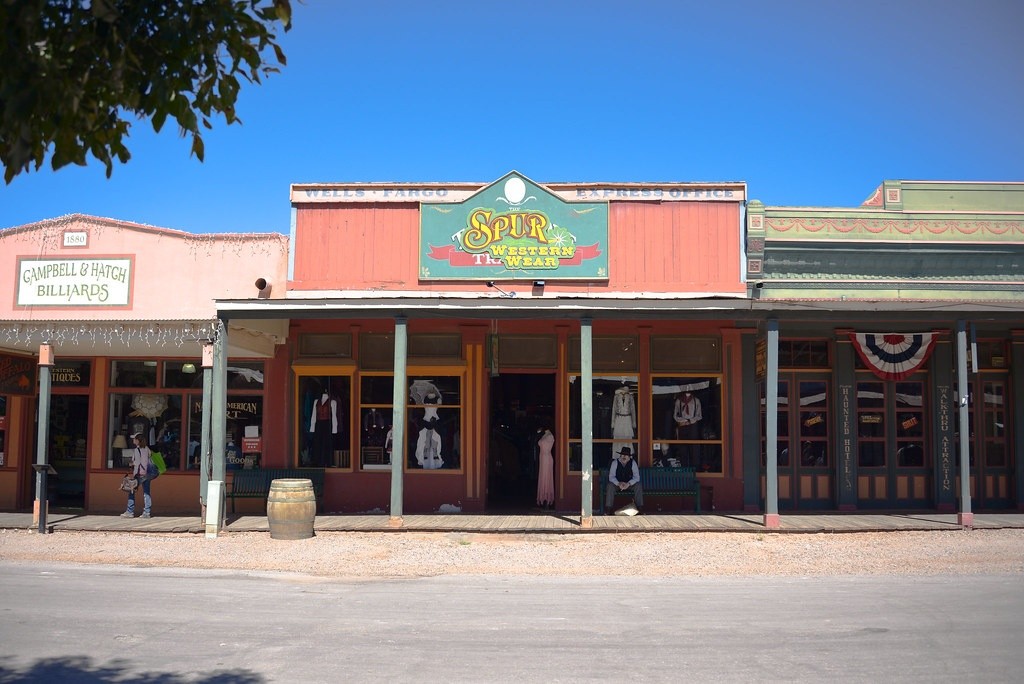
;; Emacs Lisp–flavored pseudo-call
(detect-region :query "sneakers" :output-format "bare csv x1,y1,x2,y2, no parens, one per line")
121,512,135,518
139,514,151,518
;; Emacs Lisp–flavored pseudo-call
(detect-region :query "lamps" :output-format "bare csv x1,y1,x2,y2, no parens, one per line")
182,363,196,373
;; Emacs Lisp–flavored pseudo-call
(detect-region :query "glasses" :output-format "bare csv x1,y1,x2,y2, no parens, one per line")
621,455,628,457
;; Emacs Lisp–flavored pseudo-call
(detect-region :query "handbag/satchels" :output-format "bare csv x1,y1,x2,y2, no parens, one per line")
147,451,166,482
614,499,639,516
118,474,139,494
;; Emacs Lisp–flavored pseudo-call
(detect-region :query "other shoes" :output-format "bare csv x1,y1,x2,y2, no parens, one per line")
636,507,647,516
604,507,612,516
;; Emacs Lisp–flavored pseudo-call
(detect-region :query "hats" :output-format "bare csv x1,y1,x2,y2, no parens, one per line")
130,432,142,439
616,447,634,456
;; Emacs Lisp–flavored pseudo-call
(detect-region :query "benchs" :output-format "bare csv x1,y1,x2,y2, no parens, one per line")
598,466,702,515
226,468,326,514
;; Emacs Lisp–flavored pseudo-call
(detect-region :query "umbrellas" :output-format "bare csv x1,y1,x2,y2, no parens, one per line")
130,384,169,418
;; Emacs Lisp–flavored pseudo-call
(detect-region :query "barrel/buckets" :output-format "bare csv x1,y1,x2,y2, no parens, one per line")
266,478,317,540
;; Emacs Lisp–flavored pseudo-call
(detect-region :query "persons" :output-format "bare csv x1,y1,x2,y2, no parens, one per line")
673,393,703,471
536,430,555,507
309,392,338,467
119,433,155,518
603,446,645,515
364,407,384,446
610,385,637,457
